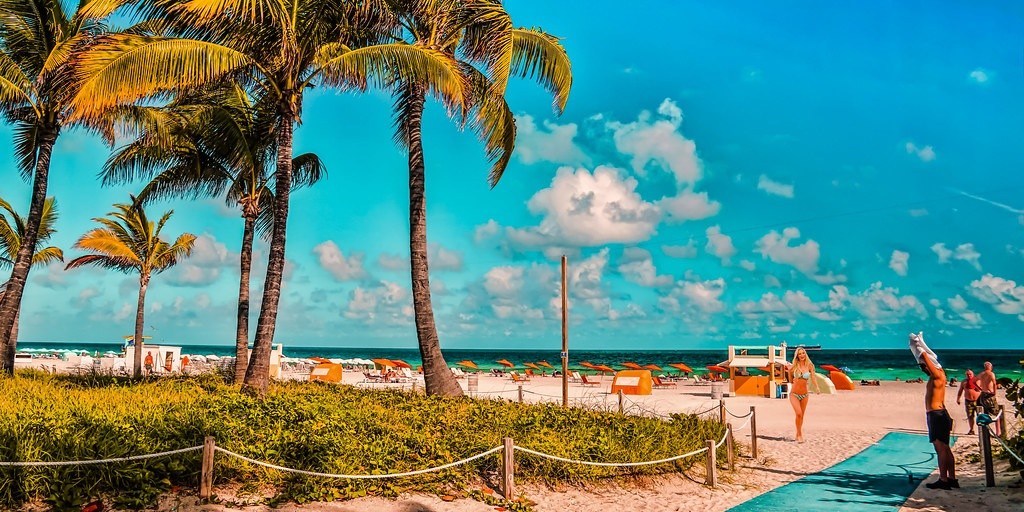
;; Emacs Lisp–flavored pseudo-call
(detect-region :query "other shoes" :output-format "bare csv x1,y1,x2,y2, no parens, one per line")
948,477,960,488
968,431,974,434
926,478,951,490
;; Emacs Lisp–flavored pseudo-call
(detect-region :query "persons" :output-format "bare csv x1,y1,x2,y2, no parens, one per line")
861,376,957,387
956,361,1001,437
385,368,396,382
665,371,689,381
542,369,561,377
788,347,820,444
494,369,507,377
144,351,154,374
909,332,960,491
702,373,723,382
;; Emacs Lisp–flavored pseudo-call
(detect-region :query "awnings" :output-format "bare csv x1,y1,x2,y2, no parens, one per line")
717,357,770,367
774,358,792,367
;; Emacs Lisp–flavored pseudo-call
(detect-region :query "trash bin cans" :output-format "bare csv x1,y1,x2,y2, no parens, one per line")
711,382,723,400
468,375,478,391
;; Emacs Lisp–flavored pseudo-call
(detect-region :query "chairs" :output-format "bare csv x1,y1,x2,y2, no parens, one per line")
363,368,724,388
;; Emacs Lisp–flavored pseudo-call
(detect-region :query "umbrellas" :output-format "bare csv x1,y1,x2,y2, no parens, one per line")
706,365,727,375
496,359,554,372
738,364,840,377
454,360,478,369
578,361,693,376
280,356,412,374
181,353,236,364
19,347,126,356
